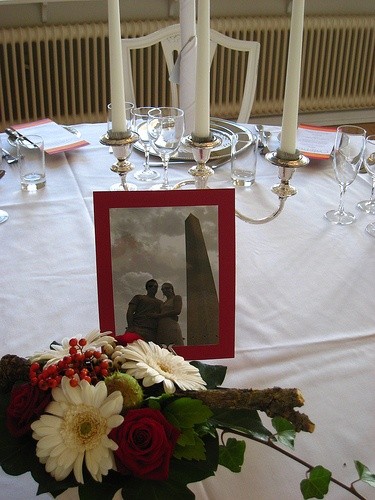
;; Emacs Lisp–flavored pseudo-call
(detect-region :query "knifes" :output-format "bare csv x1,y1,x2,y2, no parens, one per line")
4,128,34,150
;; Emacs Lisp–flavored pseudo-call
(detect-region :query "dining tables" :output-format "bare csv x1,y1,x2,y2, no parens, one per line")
0,119,375,500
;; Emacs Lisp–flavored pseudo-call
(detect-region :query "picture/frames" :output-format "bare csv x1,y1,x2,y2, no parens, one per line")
93,188,235,360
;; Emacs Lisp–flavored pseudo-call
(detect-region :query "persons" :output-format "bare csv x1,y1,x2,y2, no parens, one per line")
126,279,178,341
150,283,184,346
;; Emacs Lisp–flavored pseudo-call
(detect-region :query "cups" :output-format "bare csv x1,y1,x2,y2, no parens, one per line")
231,133,258,186
17,135,47,191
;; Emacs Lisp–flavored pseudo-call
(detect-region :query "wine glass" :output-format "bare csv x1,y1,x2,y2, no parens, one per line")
107,102,134,163
364,219,375,235
325,124,366,225
131,107,161,182
356,135,375,215
148,107,185,190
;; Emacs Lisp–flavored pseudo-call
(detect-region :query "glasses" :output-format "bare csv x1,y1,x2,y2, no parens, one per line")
161,286,172,290
148,285,157,288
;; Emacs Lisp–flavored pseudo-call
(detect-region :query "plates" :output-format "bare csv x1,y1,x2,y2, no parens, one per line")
276,131,351,153
6,126,80,149
131,116,253,160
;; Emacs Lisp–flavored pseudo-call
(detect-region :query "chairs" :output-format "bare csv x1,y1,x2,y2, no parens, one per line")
122,25,260,124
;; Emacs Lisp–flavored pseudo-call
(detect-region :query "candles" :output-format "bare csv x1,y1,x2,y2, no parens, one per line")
195,0,210,137
108,0,126,133
279,0,305,155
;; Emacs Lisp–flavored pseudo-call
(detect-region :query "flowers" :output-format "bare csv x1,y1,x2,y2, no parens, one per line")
0,330,375,500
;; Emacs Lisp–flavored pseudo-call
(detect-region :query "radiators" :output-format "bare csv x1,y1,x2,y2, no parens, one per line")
0,15,375,133
